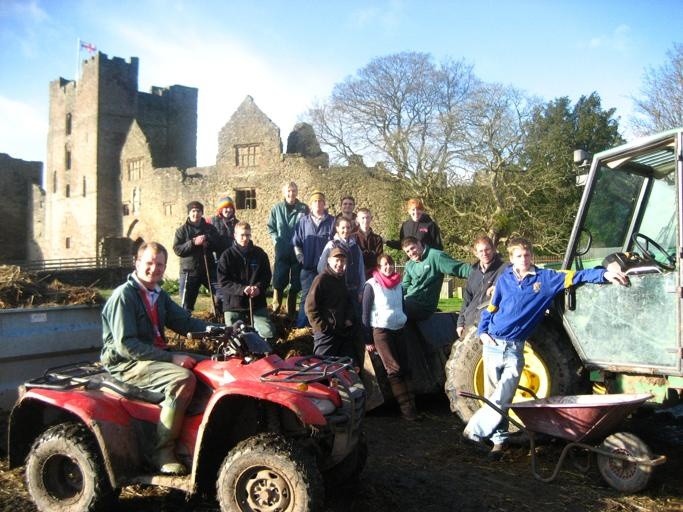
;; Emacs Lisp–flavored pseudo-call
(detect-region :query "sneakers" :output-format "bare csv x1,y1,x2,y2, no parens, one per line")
486,442,507,463
399,406,426,422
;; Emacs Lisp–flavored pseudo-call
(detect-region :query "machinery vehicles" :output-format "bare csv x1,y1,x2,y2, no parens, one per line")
445,127,683,442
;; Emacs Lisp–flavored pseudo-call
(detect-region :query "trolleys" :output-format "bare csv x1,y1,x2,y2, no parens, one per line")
459,387,667,496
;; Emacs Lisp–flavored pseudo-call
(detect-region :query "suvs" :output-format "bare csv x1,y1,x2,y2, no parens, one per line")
9,335,361,508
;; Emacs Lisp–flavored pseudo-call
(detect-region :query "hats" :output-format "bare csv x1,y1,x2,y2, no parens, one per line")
307,188,326,205
329,246,347,257
216,194,236,220
186,199,203,215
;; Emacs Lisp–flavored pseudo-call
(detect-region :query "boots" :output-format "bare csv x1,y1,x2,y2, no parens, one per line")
142,402,190,477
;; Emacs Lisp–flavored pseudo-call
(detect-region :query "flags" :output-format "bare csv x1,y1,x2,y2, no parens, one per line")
74,37,102,55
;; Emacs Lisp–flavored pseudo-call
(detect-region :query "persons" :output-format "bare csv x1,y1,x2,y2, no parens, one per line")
351,208,383,261
217,222,275,339
361,254,428,421
304,247,356,357
173,202,223,313
99,242,245,475
399,236,475,318
206,197,239,244
291,193,336,328
462,236,629,463
382,198,443,260
267,181,310,316
335,197,357,232
457,235,511,338
317,217,366,303
